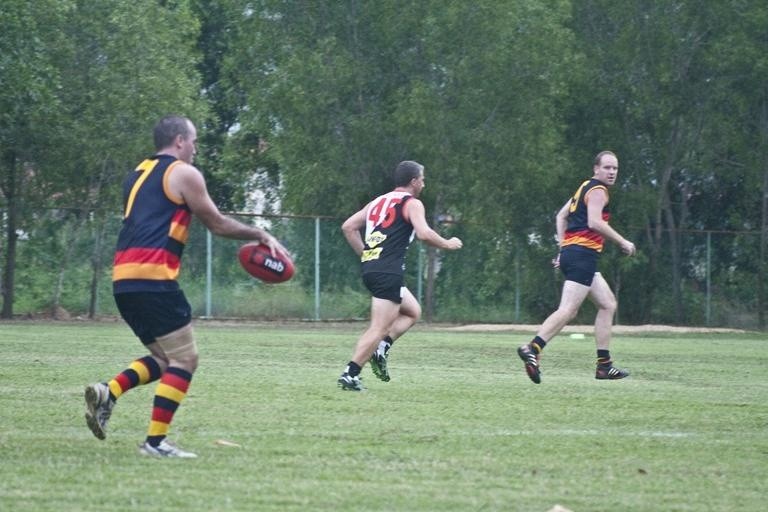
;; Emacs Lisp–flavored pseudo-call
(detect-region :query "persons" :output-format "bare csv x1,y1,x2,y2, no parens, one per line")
335,160,463,391
85,114,290,461
518,151,635,383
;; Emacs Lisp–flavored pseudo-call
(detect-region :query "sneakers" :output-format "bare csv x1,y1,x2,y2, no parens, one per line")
595,356,631,380
517,342,541,384
84,383,115,441
138,441,199,459
337,337,390,391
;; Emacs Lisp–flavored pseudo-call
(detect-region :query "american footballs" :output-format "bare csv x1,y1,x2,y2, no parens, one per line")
239,245,294,284
